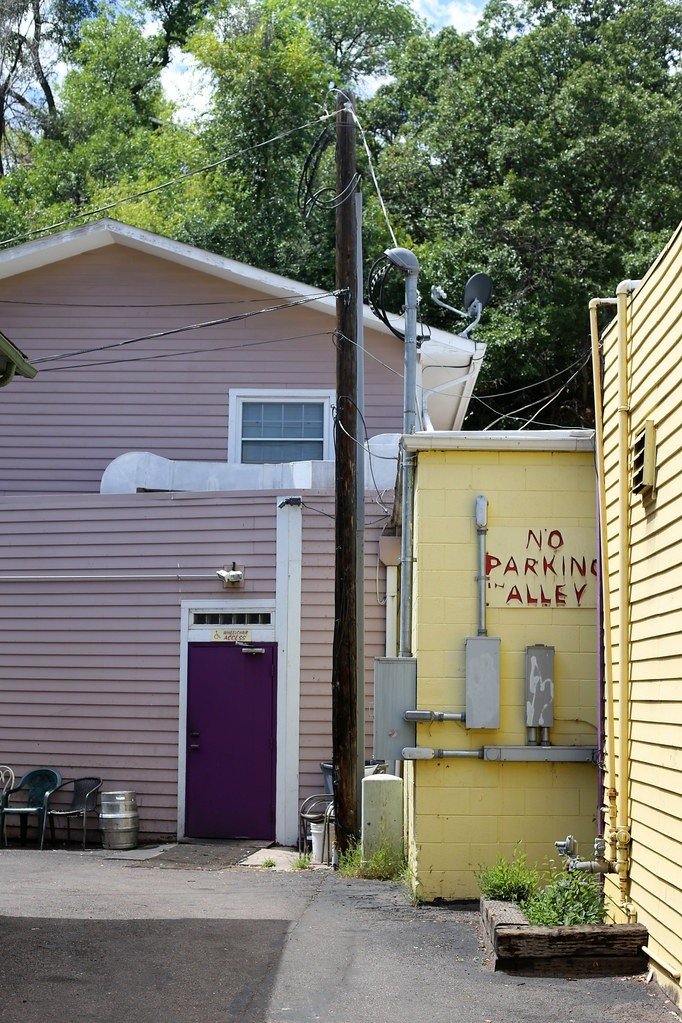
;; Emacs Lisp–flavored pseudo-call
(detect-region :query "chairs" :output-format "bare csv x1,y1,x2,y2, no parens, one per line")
299,794,340,873
0,769,62,850
41,777,103,851
0,765,14,847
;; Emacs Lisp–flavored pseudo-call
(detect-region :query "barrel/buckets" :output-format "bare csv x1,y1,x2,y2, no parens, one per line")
98,789,138,850
310,822,334,862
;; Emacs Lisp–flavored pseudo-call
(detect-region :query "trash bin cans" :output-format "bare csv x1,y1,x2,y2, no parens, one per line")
99,791,140,849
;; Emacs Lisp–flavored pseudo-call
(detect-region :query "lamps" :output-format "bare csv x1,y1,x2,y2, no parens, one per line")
216,569,243,582
